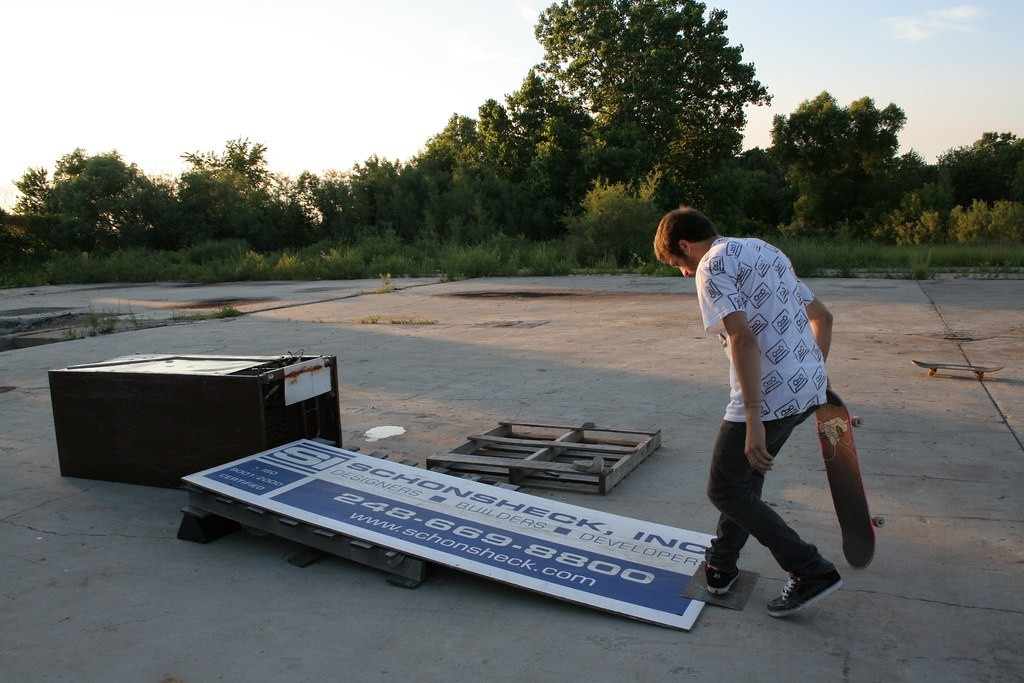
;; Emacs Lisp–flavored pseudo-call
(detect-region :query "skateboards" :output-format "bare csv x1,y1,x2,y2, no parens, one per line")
815,387,886,571
910,360,1004,380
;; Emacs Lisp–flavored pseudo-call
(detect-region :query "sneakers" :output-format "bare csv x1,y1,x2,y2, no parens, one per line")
766,563,843,617
705,563,740,595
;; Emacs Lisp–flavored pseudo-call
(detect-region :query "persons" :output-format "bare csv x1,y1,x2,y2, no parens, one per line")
653,204,843,618
